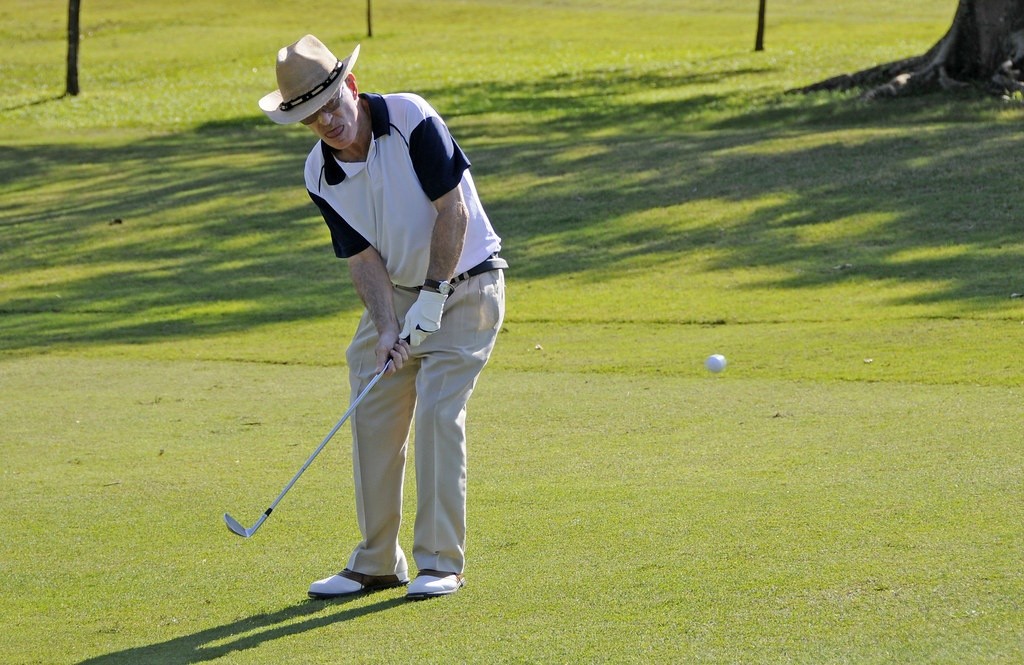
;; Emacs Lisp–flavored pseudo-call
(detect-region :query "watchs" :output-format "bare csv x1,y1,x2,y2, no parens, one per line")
424,279,455,299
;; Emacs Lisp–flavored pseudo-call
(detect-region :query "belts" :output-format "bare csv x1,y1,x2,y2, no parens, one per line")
414,252,510,291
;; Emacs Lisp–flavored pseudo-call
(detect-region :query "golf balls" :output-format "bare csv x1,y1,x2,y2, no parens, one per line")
706,354,726,371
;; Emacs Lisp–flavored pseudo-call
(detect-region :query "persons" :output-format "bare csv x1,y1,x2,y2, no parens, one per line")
260,32,509,600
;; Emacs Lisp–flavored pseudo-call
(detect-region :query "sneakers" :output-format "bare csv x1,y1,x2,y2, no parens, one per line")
406,569,466,600
307,568,411,599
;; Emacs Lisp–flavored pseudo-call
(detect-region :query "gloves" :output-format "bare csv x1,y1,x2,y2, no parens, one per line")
398,290,448,346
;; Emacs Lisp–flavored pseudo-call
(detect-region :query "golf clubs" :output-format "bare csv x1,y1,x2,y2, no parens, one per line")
224,336,412,540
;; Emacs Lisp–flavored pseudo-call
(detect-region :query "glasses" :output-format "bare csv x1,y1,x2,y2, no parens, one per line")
299,84,342,125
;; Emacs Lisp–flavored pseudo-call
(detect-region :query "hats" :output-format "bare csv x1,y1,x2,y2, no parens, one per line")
258,34,361,125
